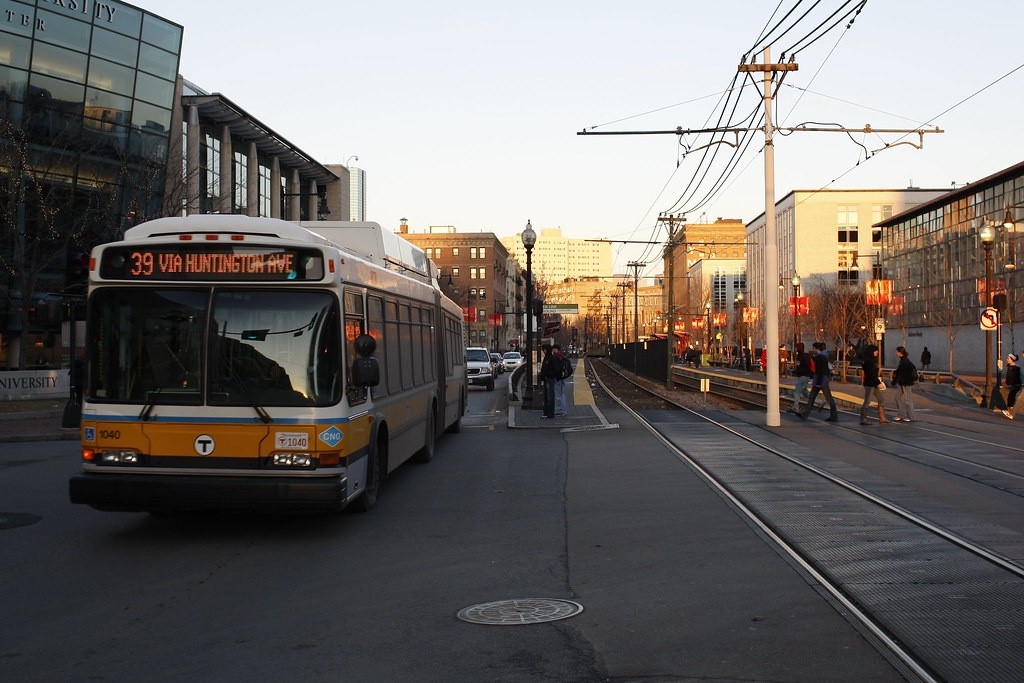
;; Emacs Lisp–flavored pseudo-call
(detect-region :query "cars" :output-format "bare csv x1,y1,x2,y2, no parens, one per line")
502,352,524,371
491,353,503,377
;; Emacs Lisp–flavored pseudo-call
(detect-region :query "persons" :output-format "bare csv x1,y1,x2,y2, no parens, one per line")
860,345,890,425
779,344,788,378
921,347,931,370
786,343,826,414
891,346,917,422
848,346,856,366
796,342,838,422
539,344,560,419
761,344,767,379
1001,351,1024,420
552,344,567,417
683,347,700,369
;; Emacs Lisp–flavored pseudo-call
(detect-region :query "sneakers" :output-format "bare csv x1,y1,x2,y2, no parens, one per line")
1002,411,1013,420
903,417,912,422
892,416,905,421
825,418,838,422
540,415,554,420
795,412,806,421
555,412,567,416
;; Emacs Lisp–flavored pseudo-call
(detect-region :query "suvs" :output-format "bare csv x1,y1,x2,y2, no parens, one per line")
466,347,494,392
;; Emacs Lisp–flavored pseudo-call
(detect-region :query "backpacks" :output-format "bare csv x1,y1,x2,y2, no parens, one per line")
826,358,839,376
808,356,816,375
558,356,572,379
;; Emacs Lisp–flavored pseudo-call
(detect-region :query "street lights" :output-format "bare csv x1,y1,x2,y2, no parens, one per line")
737,291,744,368
979,219,996,407
791,272,800,343
281,191,332,222
706,303,711,354
734,292,752,351
851,253,882,372
779,277,800,362
653,318,657,334
521,219,537,409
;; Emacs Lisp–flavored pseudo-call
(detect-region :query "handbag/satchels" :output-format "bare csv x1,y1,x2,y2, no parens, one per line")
910,362,918,381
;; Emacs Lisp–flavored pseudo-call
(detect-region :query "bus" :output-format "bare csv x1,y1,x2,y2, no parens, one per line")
67,214,467,511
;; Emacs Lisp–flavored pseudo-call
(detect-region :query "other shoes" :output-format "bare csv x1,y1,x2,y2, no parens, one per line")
786,408,796,412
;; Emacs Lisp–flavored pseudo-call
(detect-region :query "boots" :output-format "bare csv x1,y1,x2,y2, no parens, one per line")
860,408,873,425
878,406,892,423
